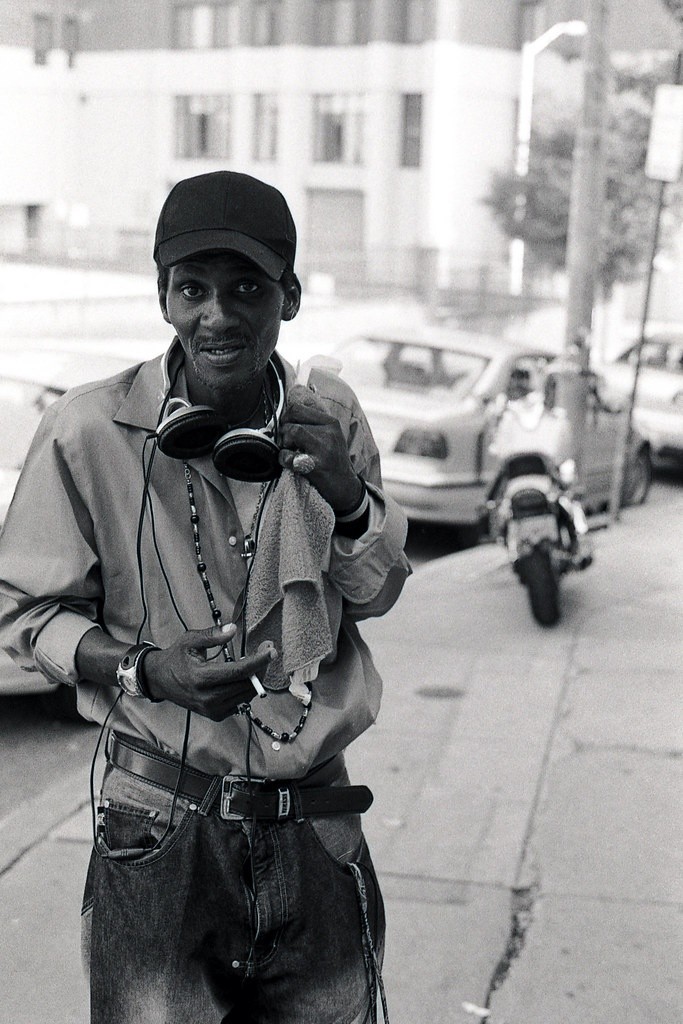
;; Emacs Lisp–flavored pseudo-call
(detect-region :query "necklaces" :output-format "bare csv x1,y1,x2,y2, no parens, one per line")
184,387,312,742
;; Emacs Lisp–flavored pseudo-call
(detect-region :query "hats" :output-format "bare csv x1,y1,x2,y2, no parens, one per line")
154,170,297,280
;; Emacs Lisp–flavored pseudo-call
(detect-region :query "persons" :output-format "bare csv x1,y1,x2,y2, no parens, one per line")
0,170,414,1024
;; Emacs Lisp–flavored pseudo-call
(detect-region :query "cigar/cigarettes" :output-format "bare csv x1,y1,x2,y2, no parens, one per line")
240,657,268,698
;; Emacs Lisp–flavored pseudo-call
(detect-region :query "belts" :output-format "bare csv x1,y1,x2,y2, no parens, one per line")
108,735,374,820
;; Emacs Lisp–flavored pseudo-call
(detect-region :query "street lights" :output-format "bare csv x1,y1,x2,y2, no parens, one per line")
508,21,590,289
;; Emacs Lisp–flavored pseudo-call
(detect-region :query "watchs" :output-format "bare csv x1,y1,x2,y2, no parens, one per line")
115,640,165,703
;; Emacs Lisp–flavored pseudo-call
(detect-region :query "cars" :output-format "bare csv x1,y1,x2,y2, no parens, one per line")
329,324,652,554
605,321,682,459
0,351,157,724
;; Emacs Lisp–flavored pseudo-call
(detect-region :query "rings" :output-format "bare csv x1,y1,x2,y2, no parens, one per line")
292,453,315,476
238,702,251,713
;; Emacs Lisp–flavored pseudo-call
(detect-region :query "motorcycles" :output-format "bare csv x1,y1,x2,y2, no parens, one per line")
472,368,593,627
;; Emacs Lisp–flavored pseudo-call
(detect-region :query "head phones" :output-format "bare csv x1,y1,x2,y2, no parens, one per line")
156,352,285,482
